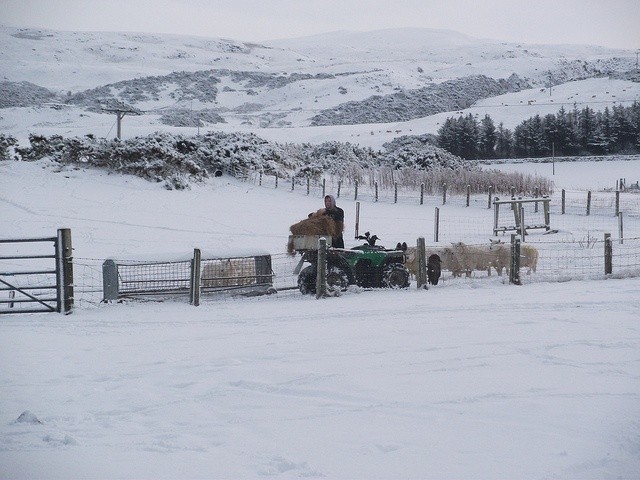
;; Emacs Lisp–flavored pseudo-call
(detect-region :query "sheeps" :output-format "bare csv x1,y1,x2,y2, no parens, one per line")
488,238,539,275
451,241,493,278
200,258,236,286
404,247,438,280
442,247,472,277
432,248,448,271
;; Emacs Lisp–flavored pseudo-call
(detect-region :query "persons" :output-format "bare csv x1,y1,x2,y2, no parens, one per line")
306,191,346,249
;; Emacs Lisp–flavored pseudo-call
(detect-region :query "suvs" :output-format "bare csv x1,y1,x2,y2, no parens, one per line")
298,231,410,296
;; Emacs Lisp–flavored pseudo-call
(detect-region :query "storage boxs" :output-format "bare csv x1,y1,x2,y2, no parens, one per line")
288,234,332,250
